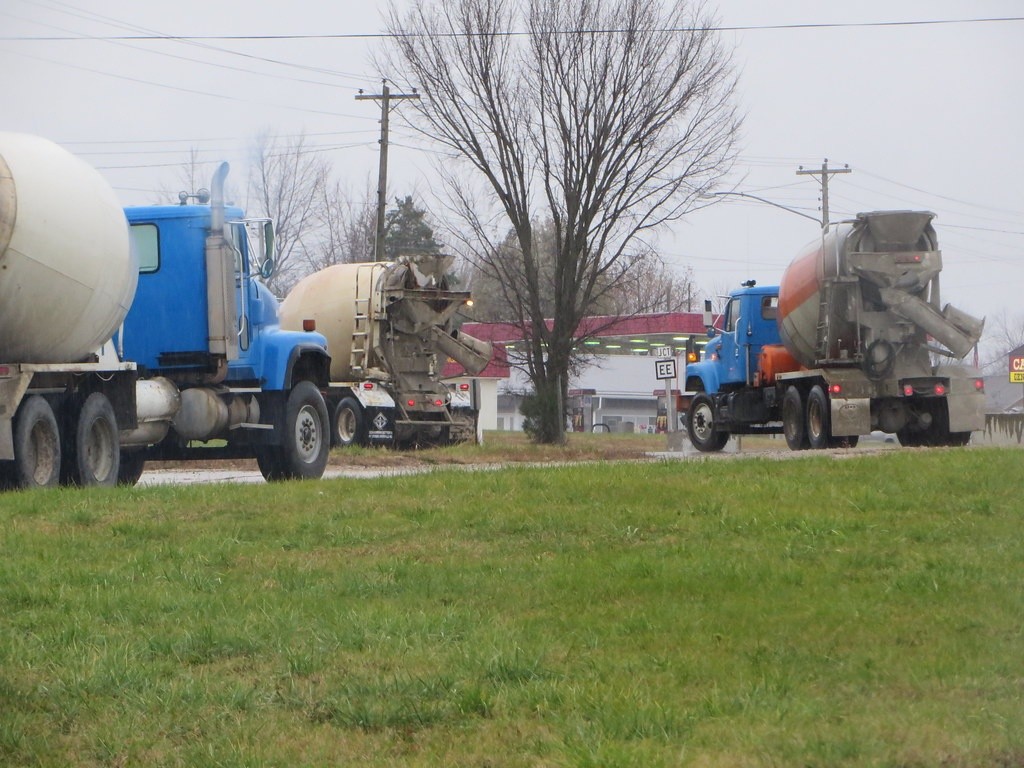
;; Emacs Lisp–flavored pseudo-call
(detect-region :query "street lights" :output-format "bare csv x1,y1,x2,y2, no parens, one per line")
702,189,831,234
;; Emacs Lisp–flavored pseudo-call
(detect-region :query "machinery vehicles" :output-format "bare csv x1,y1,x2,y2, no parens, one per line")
684,210,988,454
0,122,333,496
279,252,494,448
